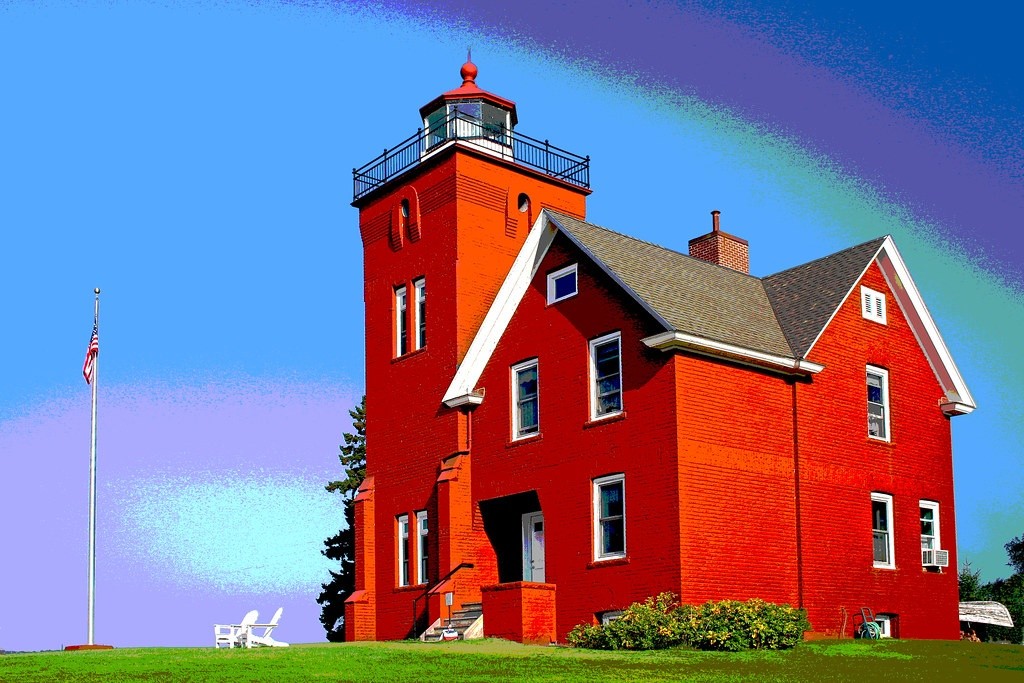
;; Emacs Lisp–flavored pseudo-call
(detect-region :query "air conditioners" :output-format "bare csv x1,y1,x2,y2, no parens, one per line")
921,548,949,567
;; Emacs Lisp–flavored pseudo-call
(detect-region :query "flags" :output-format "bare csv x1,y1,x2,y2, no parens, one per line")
82,315,99,386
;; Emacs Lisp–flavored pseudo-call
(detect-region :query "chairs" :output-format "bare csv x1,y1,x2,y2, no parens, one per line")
214,608,290,648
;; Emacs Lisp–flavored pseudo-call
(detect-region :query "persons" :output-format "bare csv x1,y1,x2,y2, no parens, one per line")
960,628,981,642
